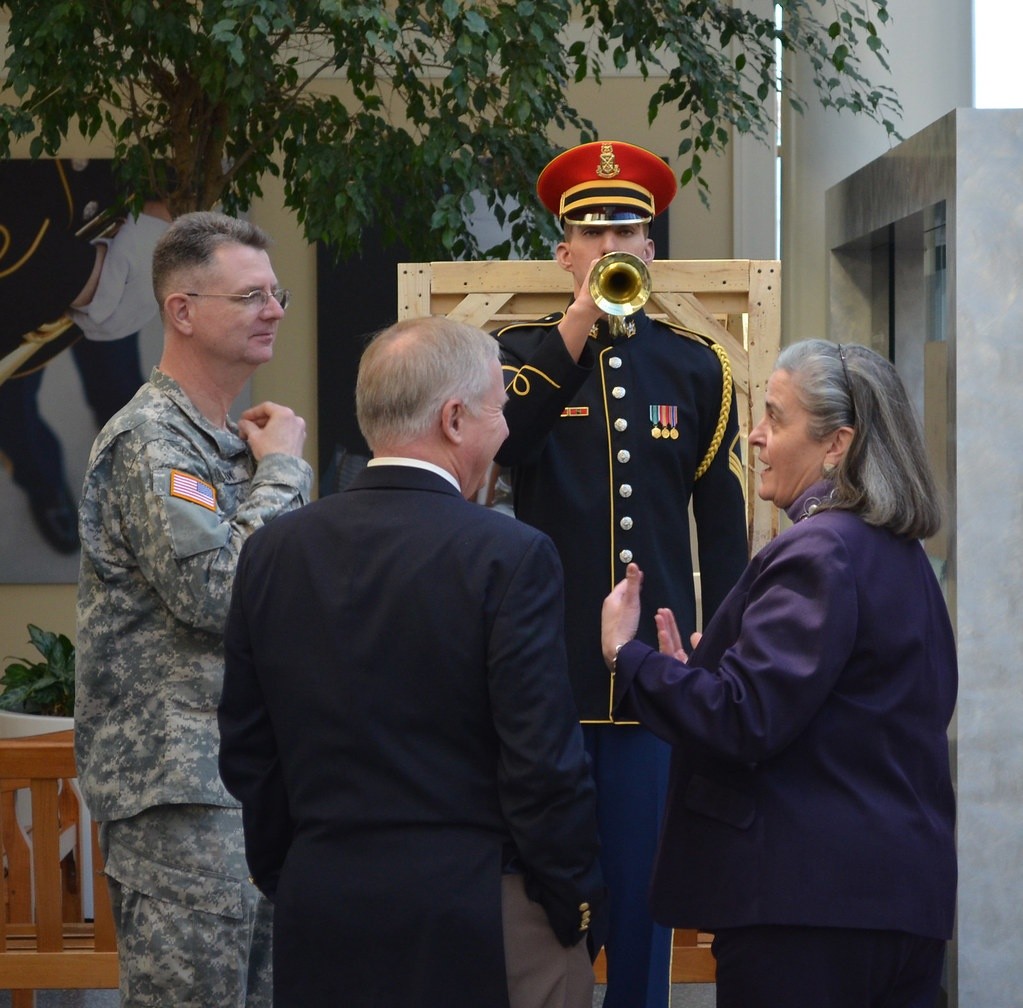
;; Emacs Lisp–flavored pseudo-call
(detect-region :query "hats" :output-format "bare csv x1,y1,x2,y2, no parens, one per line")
536,140,677,225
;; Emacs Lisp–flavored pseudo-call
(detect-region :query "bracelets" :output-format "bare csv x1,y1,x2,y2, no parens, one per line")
612,638,628,684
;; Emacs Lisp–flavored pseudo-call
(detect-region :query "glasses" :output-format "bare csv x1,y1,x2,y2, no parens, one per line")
183,288,292,313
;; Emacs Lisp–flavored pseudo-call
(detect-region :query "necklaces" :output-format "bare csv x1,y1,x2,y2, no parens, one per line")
796,486,839,523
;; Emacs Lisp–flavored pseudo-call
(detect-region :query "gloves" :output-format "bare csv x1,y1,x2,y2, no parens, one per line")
73,211,171,342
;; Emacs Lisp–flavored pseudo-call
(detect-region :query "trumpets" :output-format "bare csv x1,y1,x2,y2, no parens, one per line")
588,251,654,317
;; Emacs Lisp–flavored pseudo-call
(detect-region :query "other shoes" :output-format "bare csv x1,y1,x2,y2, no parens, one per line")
29,477,79,554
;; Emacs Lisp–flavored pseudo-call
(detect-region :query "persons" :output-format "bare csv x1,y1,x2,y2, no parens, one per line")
0,160,195,558
601,339,959,1008
57,212,322,1008
215,314,604,1008
485,139,752,1008
